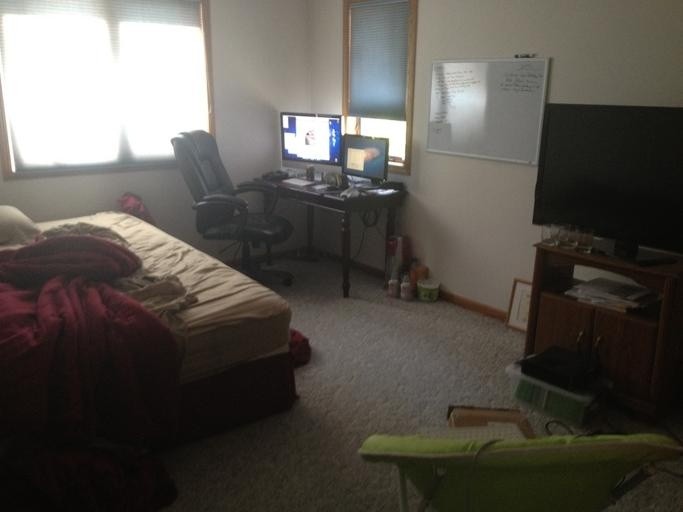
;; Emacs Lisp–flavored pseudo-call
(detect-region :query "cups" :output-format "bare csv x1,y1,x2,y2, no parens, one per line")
541,221,593,253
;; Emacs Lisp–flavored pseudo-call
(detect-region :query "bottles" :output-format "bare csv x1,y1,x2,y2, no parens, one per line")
388,270,412,300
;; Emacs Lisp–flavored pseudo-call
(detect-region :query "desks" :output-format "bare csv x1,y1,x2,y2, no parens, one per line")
254,171,409,297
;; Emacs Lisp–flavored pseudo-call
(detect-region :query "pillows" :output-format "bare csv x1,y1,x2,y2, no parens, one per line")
1,205,44,247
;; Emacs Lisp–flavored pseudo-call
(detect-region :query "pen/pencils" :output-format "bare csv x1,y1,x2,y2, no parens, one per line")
515,54,535,58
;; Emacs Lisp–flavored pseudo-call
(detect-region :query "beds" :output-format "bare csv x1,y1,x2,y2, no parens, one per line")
1,209,293,465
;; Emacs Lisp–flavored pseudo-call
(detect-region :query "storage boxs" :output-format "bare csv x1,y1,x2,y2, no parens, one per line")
507,353,594,426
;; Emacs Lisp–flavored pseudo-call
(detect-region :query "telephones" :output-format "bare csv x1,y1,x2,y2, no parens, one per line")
262,170,287,181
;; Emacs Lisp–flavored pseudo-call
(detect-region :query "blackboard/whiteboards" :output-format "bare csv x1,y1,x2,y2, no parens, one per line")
424,57,551,165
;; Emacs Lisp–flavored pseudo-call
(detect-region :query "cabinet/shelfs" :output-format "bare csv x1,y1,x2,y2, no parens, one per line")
524,237,682,426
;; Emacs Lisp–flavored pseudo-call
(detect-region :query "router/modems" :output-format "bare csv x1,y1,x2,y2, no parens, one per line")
262,171,285,181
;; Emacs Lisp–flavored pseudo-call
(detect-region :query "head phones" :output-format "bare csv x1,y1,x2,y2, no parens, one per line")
323,172,349,191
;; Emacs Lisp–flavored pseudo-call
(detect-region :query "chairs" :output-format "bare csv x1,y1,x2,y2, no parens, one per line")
357,421,683,512
170,129,294,286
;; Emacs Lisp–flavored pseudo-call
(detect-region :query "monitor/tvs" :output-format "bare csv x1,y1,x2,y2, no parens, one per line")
531,102,683,268
279,111,344,185
342,133,390,190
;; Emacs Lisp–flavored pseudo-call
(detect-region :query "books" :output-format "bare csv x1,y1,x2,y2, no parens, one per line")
564,276,662,313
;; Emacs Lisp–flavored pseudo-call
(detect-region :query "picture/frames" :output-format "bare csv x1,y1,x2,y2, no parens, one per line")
505,279,532,333
